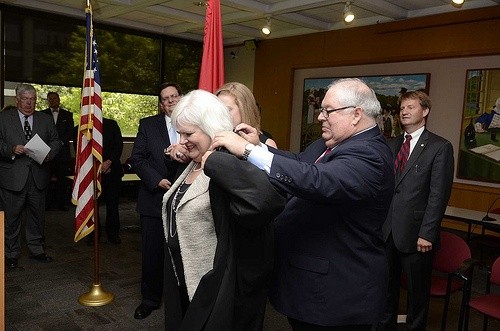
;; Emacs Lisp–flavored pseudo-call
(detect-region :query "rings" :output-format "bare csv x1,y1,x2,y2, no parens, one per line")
176,151,181,158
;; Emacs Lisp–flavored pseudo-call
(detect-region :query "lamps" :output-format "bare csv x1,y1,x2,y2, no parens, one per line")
343,1,354,23
261,13,272,35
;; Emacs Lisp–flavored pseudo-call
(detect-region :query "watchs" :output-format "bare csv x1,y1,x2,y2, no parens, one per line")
242,143,255,160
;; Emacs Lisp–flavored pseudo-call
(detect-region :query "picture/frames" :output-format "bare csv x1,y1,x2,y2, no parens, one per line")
289,52,500,195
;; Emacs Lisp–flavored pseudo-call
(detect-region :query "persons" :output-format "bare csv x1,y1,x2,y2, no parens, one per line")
208,77,396,331
158,87,290,331
132,81,183,320
305,81,326,124
389,90,455,331
376,103,396,140
214,81,279,150
0,83,124,269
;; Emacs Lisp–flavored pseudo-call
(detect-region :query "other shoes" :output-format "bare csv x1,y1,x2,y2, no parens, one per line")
87,236,95,246
108,237,121,244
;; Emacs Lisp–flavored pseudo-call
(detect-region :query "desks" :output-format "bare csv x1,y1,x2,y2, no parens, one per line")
65,174,142,185
443,206,500,270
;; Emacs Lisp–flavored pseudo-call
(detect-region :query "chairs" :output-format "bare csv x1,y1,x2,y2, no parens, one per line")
466,257,500,331
399,232,472,331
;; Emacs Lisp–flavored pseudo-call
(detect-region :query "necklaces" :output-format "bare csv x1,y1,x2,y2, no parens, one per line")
168,163,202,239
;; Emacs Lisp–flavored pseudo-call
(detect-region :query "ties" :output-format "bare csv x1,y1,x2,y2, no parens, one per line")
169,126,178,145
53,111,57,125
23,115,32,141
393,135,412,177
315,149,331,164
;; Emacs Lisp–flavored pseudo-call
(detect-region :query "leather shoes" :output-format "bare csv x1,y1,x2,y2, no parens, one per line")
61,204,70,211
133,303,160,319
6,258,16,268
29,254,53,262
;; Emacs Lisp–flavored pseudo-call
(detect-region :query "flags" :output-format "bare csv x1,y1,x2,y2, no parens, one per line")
197,0,225,93
70,3,103,243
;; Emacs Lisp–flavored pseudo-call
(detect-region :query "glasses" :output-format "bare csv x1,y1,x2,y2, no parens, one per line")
319,106,356,118
